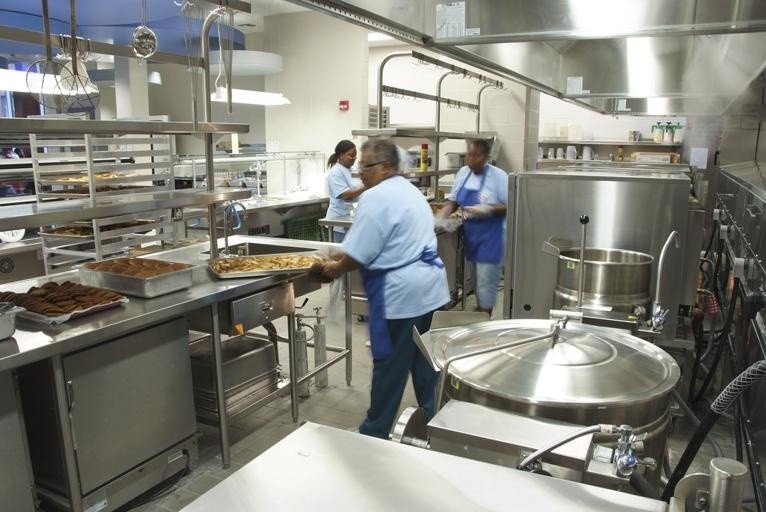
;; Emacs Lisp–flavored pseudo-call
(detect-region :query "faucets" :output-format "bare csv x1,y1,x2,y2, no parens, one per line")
224,202,249,254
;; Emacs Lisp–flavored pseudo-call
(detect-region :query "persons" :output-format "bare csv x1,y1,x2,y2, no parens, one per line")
432,140,508,316
325,140,364,242
322,138,450,438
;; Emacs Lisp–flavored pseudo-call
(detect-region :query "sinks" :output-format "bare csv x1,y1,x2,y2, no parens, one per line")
202,243,318,256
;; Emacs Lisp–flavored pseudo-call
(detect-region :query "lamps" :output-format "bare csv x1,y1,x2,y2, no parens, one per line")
210,19,291,106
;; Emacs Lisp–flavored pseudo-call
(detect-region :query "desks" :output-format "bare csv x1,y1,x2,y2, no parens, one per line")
177,422,669,512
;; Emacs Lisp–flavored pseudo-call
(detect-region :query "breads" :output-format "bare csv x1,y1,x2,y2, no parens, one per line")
85,258,190,279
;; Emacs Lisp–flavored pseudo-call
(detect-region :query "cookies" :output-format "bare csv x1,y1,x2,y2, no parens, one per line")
0,278,122,317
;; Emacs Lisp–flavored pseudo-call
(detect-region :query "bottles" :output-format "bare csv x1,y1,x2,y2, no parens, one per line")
617,145,624,161
421,144,428,172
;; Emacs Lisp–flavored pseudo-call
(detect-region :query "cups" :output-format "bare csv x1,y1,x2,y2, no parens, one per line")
538,146,594,160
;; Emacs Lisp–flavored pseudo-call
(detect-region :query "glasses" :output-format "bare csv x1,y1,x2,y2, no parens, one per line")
356,161,386,169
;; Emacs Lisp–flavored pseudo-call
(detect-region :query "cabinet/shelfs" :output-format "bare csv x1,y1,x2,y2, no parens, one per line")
19,315,198,512
539,139,690,172
0,117,252,289
353,128,497,202
